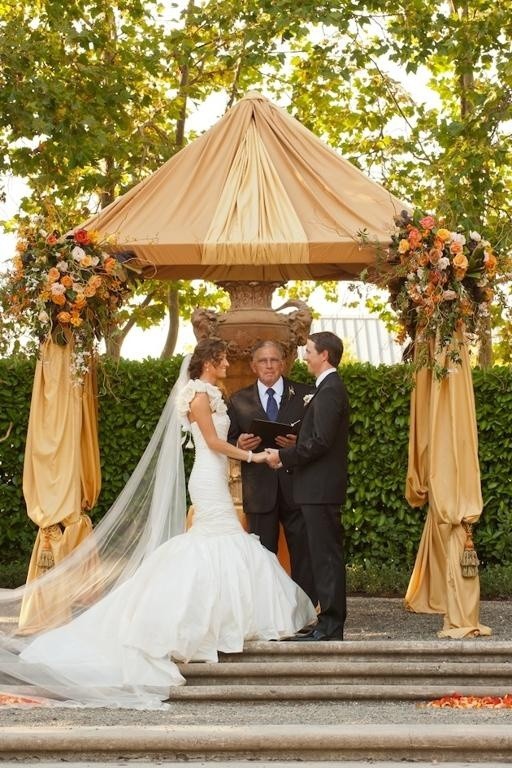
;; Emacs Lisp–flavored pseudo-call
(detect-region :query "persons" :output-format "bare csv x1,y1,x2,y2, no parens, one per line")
265,332,350,641
178,336,279,642
227,339,317,608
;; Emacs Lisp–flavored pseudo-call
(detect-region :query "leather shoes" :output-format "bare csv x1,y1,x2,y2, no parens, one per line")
292,626,344,641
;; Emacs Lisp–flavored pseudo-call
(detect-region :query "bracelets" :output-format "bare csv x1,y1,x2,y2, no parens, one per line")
247,451,253,464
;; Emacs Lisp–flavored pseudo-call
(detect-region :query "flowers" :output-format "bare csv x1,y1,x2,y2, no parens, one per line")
1,212,130,395
356,192,502,381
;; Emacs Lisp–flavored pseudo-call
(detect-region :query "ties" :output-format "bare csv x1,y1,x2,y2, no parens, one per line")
265,388,279,424
